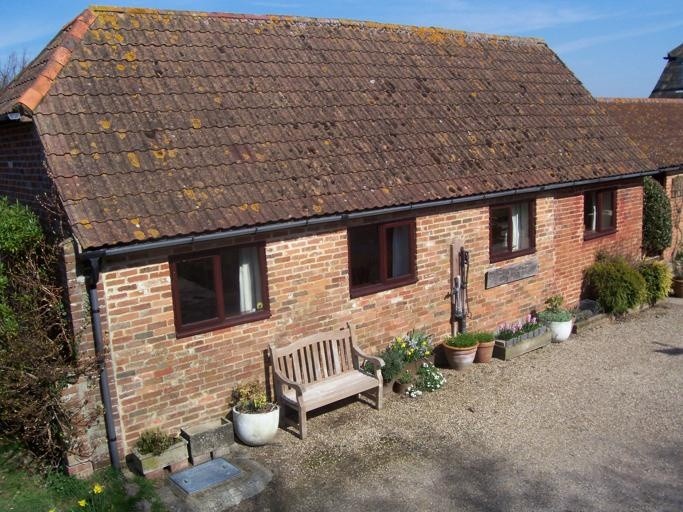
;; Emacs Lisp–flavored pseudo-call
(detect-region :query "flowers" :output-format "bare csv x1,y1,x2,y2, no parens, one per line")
497,314,537,335
360,328,446,397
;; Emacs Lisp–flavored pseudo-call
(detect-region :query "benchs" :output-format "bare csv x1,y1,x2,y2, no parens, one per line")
267,320,386,439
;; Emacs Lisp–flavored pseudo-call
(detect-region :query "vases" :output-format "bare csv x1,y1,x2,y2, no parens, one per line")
181,416,234,457
492,322,551,361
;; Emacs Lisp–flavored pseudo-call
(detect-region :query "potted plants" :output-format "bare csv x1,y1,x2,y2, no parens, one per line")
443,333,479,368
132,425,190,475
477,329,495,363
537,296,573,343
229,379,281,447
